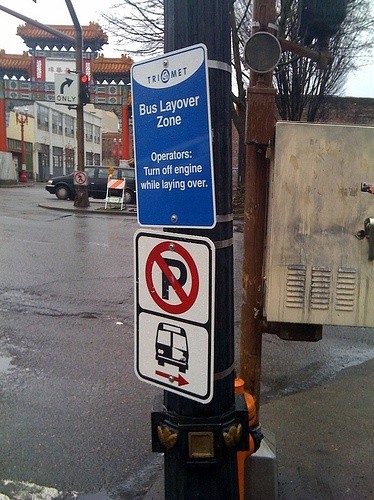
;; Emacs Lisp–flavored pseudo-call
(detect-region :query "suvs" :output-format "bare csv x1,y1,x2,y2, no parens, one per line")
45,165,136,205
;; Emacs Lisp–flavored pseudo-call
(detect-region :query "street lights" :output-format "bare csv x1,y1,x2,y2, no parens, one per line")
14,108,29,182
113,138,121,167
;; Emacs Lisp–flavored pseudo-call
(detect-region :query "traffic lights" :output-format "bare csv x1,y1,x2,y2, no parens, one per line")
78,74,91,105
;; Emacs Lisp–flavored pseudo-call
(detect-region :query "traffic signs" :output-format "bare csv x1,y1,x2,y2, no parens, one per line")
54,73,79,106
